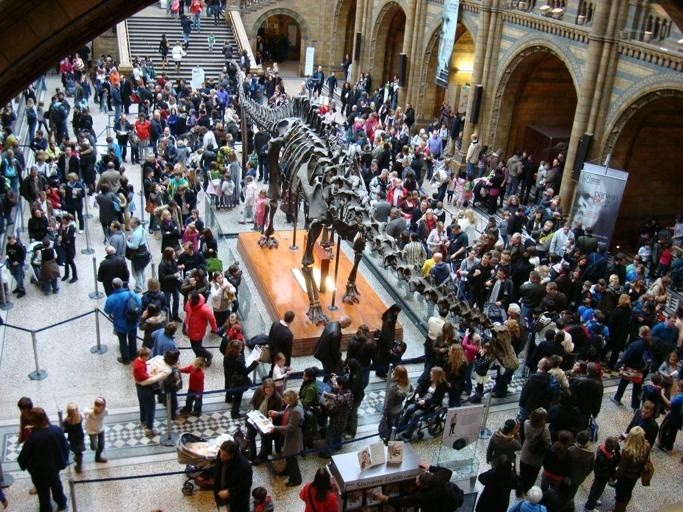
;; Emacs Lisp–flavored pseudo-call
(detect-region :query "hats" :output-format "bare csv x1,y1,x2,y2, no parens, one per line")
526,485,544,504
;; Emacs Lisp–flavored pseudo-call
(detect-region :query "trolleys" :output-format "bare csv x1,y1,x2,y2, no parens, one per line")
65,70,75,97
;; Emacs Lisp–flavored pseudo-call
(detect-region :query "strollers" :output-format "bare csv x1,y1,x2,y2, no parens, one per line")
173,422,265,497
27,239,58,288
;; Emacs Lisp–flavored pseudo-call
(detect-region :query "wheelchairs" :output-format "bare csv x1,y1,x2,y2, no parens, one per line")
404,384,451,441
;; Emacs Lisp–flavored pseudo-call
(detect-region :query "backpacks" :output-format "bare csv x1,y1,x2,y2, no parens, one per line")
125,290,142,325
547,373,560,402
637,340,654,371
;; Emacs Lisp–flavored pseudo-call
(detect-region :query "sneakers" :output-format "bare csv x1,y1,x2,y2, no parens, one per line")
117,317,216,365
225,397,244,418
585,477,617,512
244,431,358,487
610,394,620,405
26,457,113,510
12,277,80,299
137,408,209,440
450,386,512,408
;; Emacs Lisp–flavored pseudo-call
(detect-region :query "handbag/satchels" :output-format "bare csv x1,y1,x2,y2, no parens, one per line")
124,248,136,260
528,439,546,455
618,367,644,384
303,410,359,437
203,251,223,272
641,460,653,488
227,372,253,394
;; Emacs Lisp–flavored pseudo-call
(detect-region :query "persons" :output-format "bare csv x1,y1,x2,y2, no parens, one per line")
1,0,682,512
